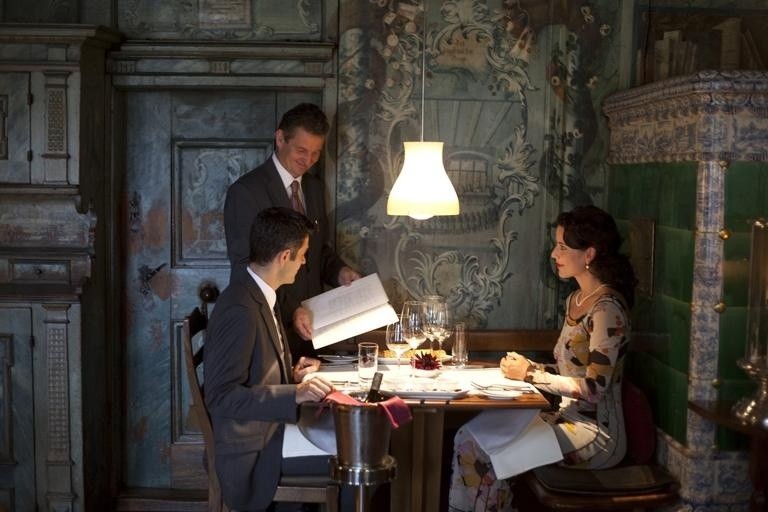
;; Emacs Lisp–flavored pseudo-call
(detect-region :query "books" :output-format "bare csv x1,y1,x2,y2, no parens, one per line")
298,271,397,352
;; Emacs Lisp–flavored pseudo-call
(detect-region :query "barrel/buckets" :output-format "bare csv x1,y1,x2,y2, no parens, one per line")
332,389,397,468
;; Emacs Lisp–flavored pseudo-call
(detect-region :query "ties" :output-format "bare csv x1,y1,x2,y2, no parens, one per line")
290,181,305,216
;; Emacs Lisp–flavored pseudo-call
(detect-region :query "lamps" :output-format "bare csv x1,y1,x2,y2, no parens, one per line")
386,4,462,221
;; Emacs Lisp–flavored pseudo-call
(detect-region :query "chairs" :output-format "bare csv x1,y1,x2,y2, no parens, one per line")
183,304,338,511
522,375,678,509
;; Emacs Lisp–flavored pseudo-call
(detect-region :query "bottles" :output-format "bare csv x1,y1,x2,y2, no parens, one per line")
356,371,384,404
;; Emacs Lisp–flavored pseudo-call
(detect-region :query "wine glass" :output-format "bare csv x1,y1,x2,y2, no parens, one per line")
353,292,469,397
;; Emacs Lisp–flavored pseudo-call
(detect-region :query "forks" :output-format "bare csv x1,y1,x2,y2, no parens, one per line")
470,381,534,395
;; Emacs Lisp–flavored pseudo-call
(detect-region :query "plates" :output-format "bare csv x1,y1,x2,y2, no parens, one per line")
475,385,522,401
316,351,360,367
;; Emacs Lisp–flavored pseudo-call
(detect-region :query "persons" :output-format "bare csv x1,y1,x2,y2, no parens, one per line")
576,283,611,308
445,202,642,511
201,205,383,510
222,102,363,366
